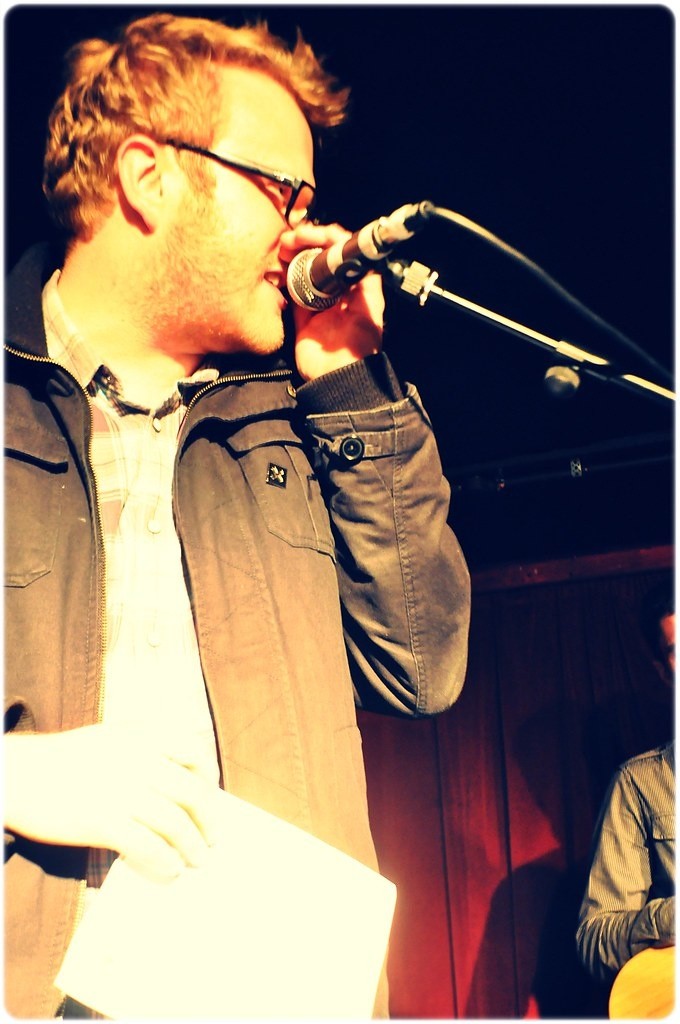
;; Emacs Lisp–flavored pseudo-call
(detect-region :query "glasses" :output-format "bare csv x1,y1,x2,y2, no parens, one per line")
154,137,317,233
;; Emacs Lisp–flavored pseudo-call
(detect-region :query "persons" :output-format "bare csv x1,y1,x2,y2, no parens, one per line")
575,584,675,1019
2,13,471,1021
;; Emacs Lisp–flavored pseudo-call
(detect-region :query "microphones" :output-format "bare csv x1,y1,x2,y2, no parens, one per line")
286,201,434,313
